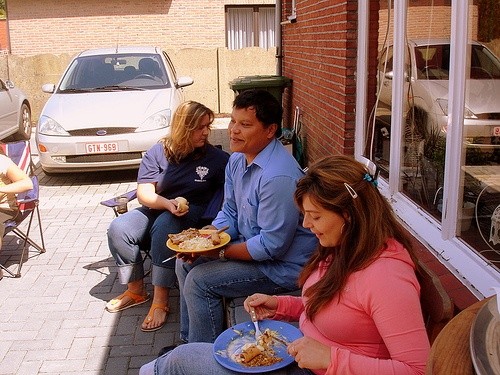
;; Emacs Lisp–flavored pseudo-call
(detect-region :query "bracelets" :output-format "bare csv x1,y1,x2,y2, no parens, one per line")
219,243,232,262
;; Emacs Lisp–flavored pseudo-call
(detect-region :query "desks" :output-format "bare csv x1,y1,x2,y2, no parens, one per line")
462,165,500,263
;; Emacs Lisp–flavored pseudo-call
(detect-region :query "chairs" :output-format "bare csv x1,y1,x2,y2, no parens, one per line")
100,188,180,292
139,58,164,84
0,140,46,277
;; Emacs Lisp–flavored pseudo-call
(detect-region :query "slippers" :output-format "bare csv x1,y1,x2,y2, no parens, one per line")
140,302,171,332
106,289,151,313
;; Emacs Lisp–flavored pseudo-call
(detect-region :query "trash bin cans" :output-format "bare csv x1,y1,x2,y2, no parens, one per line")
227,74,294,107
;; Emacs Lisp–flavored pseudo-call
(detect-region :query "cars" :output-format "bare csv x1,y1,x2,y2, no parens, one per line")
375,37,500,163
0,78,32,142
33,45,194,176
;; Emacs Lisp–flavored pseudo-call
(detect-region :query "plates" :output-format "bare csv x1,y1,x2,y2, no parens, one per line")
213,320,303,373
166,229,231,255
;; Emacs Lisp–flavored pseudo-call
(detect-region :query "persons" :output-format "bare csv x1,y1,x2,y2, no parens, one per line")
139,154,431,375
104,101,231,333
158,88,320,357
0,145,34,279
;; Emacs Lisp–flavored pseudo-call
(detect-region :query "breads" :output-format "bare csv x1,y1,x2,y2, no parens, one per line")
175,197,189,212
211,232,221,245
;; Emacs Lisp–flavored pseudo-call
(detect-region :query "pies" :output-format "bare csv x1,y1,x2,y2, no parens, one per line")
240,344,261,363
167,227,201,244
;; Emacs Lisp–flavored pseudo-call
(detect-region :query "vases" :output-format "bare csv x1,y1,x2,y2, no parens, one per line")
462,202,475,231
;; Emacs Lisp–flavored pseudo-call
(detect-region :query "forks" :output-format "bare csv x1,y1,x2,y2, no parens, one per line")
247,294,262,339
